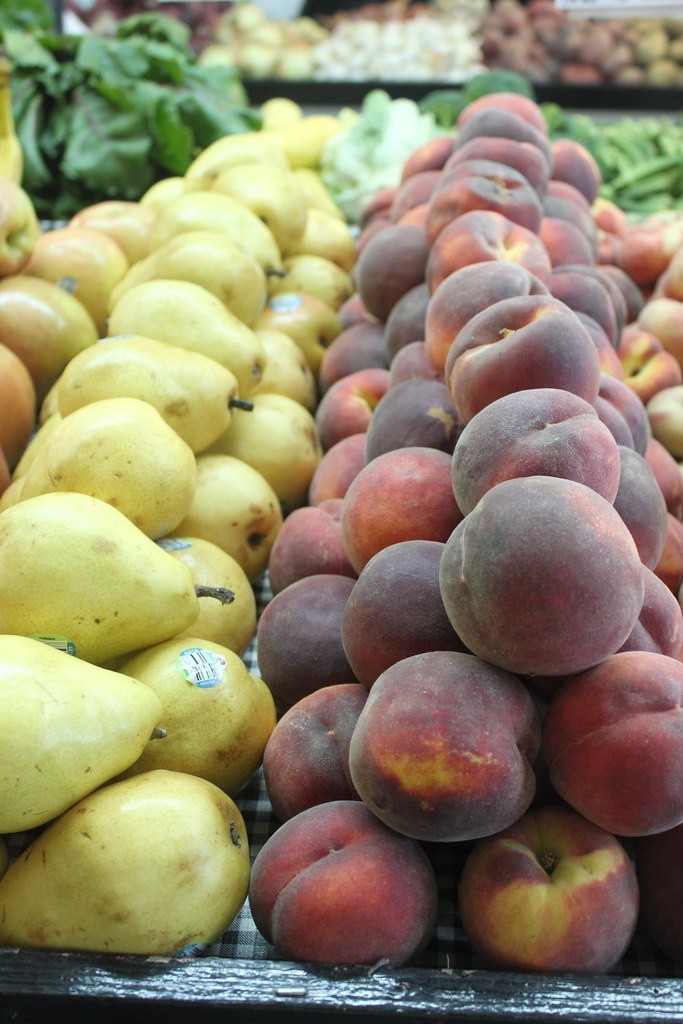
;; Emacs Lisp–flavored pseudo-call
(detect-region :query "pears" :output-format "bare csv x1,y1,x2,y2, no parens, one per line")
1,97,352,948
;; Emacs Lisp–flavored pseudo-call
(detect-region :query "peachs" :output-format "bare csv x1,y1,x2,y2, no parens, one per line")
249,87,683,971
